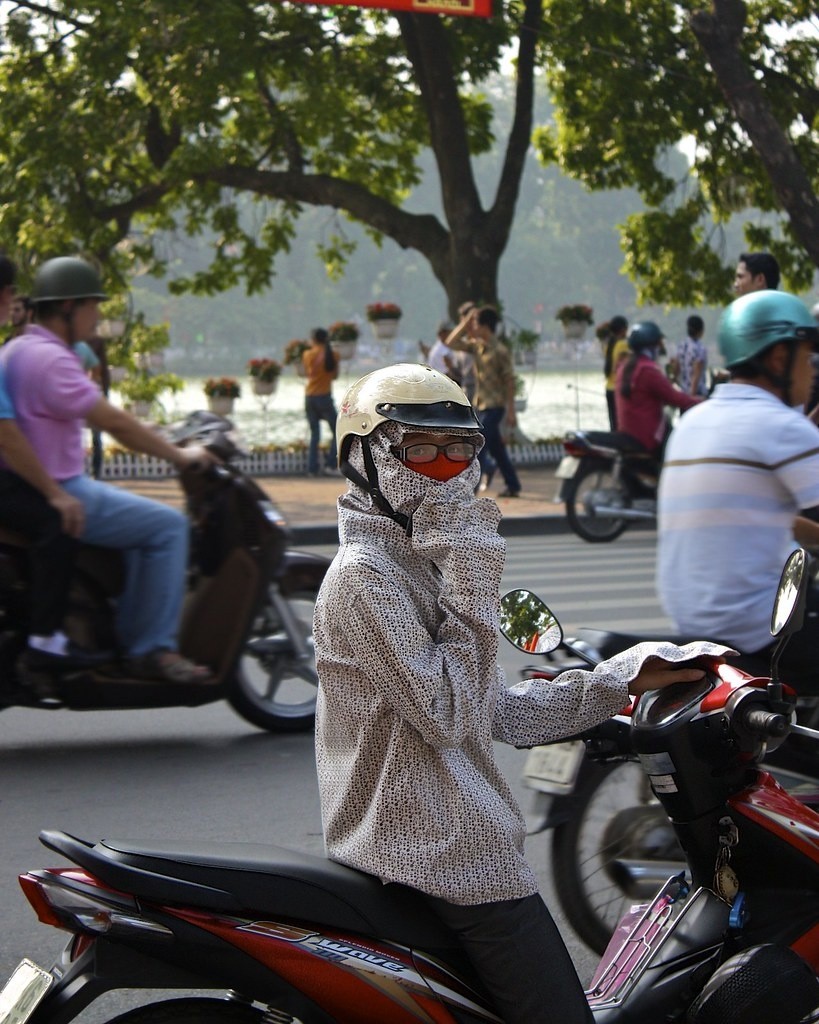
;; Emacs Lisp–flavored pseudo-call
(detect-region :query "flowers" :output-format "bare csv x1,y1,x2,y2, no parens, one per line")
365,300,403,324
554,303,595,326
326,321,361,344
203,376,241,400
284,340,312,365
248,357,284,384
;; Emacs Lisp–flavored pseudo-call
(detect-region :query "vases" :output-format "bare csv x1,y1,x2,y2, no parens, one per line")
565,320,587,337
295,361,308,377
209,395,233,414
372,318,397,338
330,341,354,359
254,378,276,394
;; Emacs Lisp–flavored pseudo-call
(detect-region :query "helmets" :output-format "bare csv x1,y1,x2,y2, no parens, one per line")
29,256,109,304
715,288,819,370
627,321,665,351
334,363,484,471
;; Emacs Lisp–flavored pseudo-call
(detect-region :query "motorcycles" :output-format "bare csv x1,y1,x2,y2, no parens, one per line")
509,556,819,965
0,408,340,746
550,423,660,544
0,546,819,1023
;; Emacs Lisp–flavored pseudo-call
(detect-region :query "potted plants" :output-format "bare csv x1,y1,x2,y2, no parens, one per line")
109,371,183,416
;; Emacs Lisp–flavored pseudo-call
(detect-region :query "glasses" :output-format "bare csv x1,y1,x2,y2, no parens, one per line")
393,441,476,463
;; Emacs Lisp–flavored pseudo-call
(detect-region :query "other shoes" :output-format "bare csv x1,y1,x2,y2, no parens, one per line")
499,487,521,497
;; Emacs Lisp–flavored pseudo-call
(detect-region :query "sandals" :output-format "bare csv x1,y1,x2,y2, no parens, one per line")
131,649,207,682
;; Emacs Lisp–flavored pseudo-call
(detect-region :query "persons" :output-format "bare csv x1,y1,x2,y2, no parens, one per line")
617,321,707,451
443,301,521,497
71,341,101,383
301,328,342,475
0,256,86,677
5,297,35,343
0,254,225,678
654,289,819,694
600,315,631,432
670,315,707,414
315,363,741,1024
733,254,779,296
428,321,459,390
